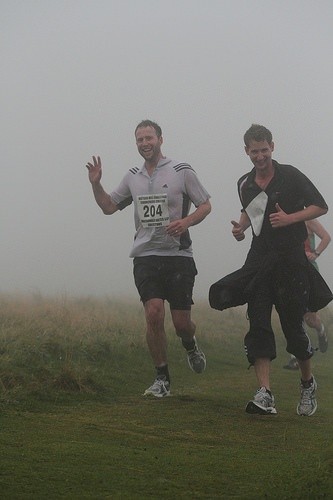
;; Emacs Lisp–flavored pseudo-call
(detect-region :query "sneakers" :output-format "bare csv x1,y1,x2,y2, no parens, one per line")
245,387,276,414
296,375,318,417
184,335,206,374
143,374,171,398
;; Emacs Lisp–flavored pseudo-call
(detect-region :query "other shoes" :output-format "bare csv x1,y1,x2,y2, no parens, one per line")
284,347,315,370
317,329,327,352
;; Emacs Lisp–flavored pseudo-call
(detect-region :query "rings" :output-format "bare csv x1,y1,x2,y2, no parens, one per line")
175,230,178,233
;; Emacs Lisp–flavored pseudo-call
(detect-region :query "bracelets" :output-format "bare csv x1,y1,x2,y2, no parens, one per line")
314,250,320,256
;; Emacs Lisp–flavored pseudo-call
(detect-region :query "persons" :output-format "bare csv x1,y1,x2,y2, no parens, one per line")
230,123,330,418
84,121,213,399
